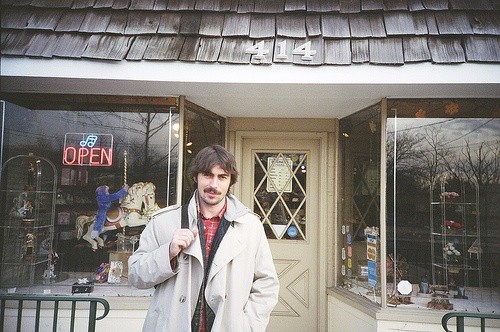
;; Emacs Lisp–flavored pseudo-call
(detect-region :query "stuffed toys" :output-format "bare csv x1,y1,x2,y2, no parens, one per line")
90,185,129,239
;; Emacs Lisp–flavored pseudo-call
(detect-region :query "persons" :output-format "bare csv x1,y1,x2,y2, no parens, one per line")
128,145,279,332
